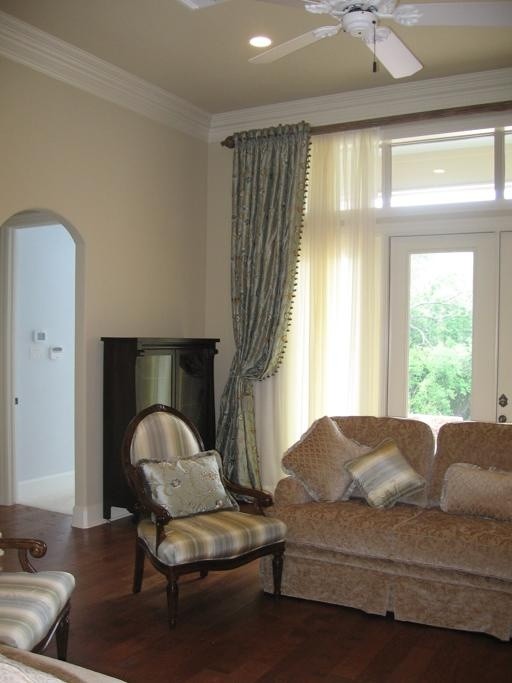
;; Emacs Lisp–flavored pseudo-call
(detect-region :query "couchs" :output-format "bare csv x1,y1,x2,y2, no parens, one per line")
259,416,512,642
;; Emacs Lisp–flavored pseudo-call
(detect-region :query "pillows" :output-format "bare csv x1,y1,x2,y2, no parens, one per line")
439,462,512,521
136,449,241,524
279,415,374,503
343,436,426,511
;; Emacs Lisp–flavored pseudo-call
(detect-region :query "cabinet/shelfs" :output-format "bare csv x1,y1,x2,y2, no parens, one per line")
100,336,221,520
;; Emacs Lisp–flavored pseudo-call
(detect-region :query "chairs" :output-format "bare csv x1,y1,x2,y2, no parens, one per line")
120,403,288,631
0,644,128,683
0,532,77,662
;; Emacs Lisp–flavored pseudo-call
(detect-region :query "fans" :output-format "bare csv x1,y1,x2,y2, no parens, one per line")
247,0,512,81
